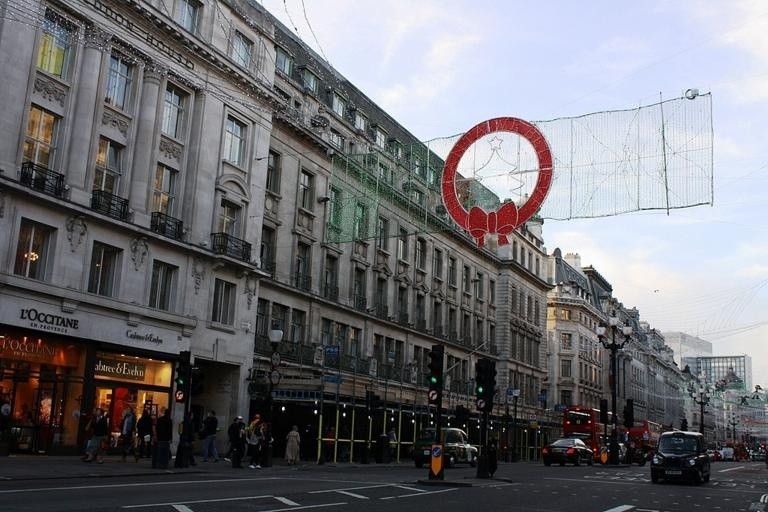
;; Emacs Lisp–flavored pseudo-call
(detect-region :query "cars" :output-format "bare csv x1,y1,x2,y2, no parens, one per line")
541,437,595,467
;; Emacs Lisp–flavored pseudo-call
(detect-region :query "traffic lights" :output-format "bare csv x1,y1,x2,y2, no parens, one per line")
427,344,444,405
174,349,192,403
474,357,494,412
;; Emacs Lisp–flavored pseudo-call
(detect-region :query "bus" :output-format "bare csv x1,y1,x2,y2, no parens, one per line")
562,405,663,461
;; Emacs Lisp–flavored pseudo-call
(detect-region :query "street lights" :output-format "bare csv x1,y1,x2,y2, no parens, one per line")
258,325,284,468
689,384,711,435
596,308,634,466
512,385,521,464
727,411,740,443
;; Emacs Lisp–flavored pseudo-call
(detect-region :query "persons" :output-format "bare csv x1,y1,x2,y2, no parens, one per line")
80,406,264,468
284,425,300,465
325,427,335,460
389,427,398,457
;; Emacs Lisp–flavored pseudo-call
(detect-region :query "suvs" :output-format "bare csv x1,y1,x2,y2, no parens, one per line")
650,430,711,487
411,428,480,468
